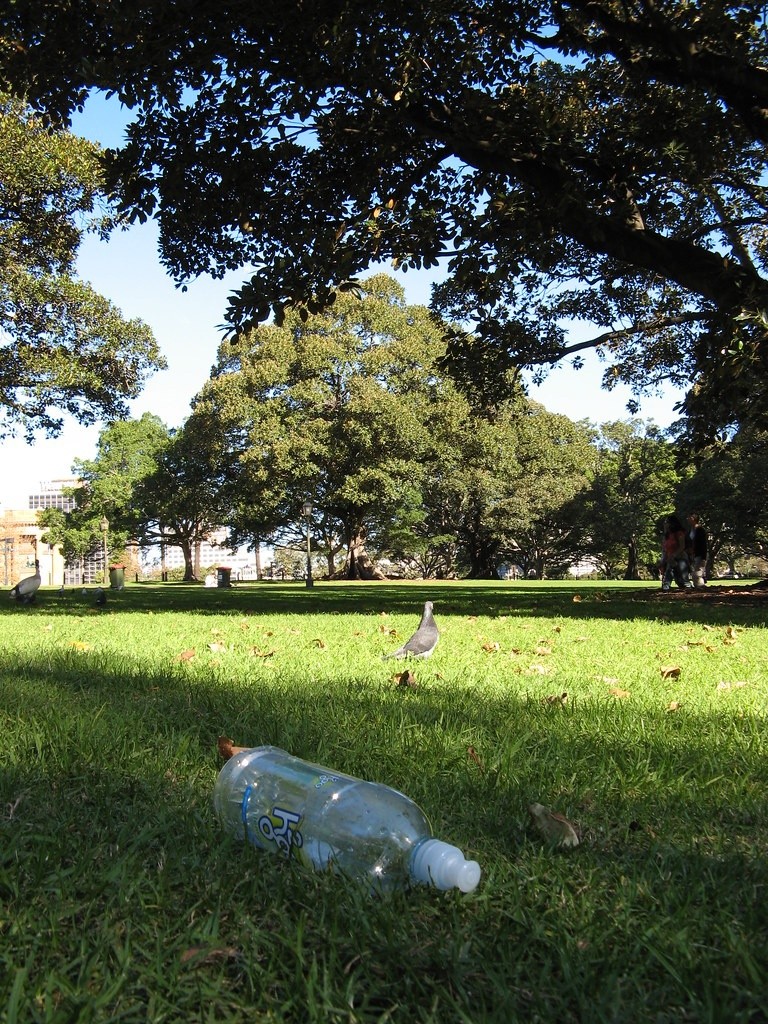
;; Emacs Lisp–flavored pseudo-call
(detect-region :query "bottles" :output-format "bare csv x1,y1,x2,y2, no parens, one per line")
215,745,481,899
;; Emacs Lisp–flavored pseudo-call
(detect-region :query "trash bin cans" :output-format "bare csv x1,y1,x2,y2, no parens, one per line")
216,567,232,588
108,564,126,589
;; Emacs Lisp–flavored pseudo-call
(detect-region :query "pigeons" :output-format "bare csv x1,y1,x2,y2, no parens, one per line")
385,601,439,663
9,559,42,601
